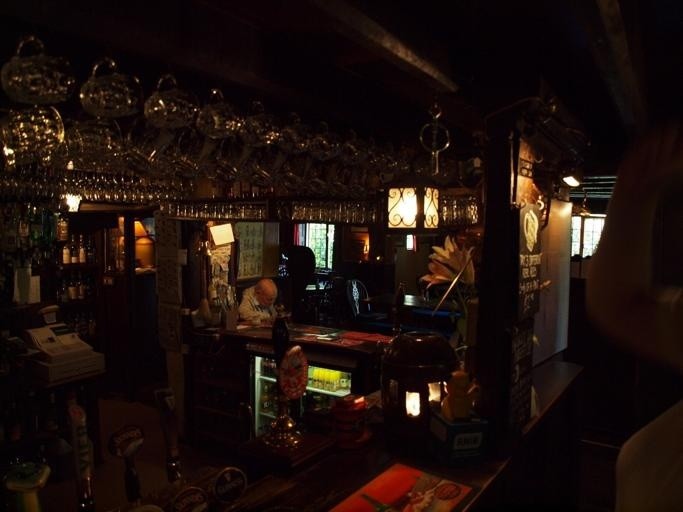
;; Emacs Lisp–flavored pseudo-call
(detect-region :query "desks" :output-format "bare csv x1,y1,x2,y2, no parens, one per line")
0,367,107,482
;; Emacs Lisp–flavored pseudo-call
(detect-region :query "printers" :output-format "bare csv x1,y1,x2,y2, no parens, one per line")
23,323,105,382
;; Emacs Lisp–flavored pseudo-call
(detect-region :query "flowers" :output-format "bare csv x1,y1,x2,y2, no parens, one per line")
420,234,480,372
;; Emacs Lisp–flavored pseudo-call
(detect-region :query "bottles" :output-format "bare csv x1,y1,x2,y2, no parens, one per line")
78,473,95,512
124,458,141,512
166,448,182,499
58,234,96,302
261,357,281,416
308,365,351,393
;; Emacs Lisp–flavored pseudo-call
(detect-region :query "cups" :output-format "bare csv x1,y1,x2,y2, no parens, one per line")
0,34,484,229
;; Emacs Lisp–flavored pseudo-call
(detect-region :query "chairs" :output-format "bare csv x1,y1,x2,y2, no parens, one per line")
297,271,406,337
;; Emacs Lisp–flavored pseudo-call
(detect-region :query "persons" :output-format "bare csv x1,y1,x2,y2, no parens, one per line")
236,278,285,325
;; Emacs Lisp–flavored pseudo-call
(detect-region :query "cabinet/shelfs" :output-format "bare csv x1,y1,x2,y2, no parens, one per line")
55,208,105,335
